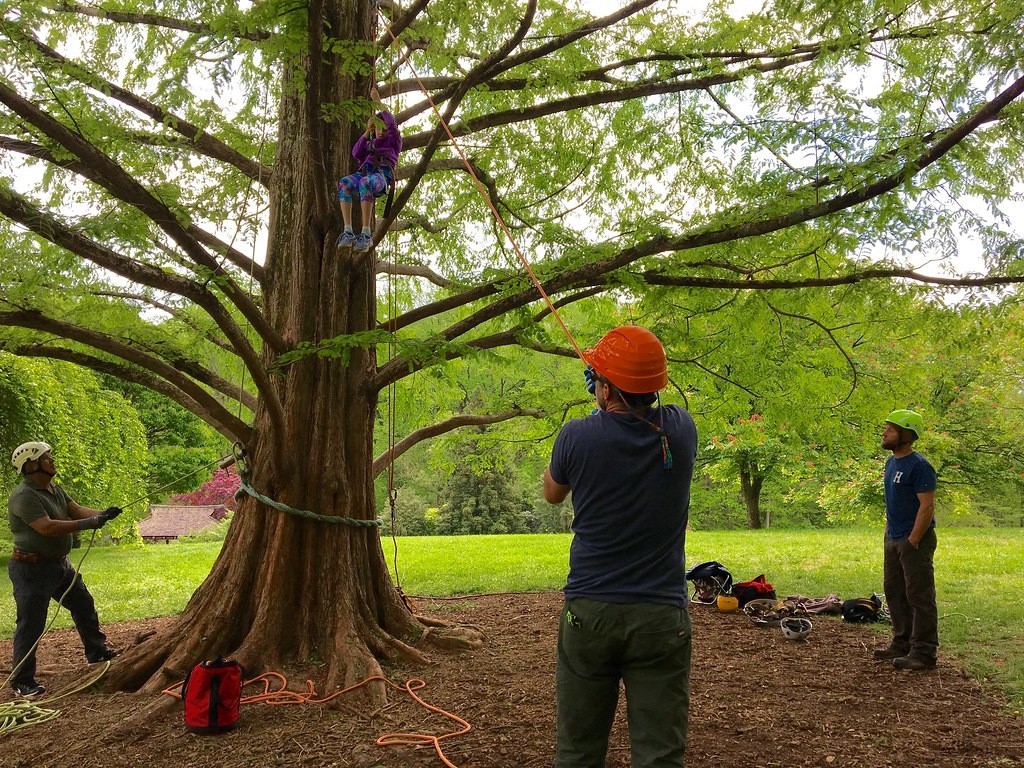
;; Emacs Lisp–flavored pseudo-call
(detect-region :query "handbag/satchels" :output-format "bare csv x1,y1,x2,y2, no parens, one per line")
731,574,776,608
181,656,243,736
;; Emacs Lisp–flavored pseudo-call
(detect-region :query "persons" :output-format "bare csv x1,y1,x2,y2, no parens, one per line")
543,325,697,768
336,89,402,253
874,409,937,670
8,442,123,697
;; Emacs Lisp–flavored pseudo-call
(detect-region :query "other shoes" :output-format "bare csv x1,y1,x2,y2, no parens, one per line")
891,656,930,670
873,648,907,658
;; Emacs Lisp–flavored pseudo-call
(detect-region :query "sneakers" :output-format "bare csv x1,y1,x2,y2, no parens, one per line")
337,231,373,253
10,680,45,697
88,648,121,667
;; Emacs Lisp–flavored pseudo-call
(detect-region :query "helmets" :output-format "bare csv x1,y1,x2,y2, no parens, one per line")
885,409,924,440
582,326,667,394
717,594,738,612
12,442,53,474
780,617,812,639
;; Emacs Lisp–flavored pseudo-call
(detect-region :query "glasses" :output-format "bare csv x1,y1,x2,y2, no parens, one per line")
589,368,605,385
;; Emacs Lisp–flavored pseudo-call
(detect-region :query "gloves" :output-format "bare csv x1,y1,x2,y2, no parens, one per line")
76,515,108,530
98,506,123,520
